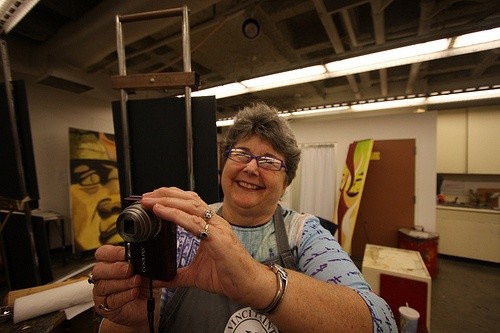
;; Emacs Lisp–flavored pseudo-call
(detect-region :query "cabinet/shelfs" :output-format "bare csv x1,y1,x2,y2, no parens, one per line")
433,104,500,264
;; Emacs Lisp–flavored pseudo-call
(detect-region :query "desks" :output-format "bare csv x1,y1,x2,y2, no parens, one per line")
34,211,66,252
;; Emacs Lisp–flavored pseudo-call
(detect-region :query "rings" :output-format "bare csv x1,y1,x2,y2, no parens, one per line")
88,272,97,285
203,209,214,221
197,223,210,241
99,297,111,311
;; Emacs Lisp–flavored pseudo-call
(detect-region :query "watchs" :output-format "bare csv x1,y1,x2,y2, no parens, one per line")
250,263,288,318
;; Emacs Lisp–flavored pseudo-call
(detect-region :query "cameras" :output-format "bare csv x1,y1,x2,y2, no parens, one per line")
116,194,177,280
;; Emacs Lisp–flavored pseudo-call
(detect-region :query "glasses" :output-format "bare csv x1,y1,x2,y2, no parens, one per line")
223,147,288,172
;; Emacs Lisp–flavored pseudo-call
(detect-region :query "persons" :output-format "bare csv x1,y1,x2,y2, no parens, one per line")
87,99,399,333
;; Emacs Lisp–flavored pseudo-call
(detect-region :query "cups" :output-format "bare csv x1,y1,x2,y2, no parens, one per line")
399,306,420,333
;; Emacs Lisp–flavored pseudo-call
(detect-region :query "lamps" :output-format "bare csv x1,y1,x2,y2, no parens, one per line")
240,18,261,39
